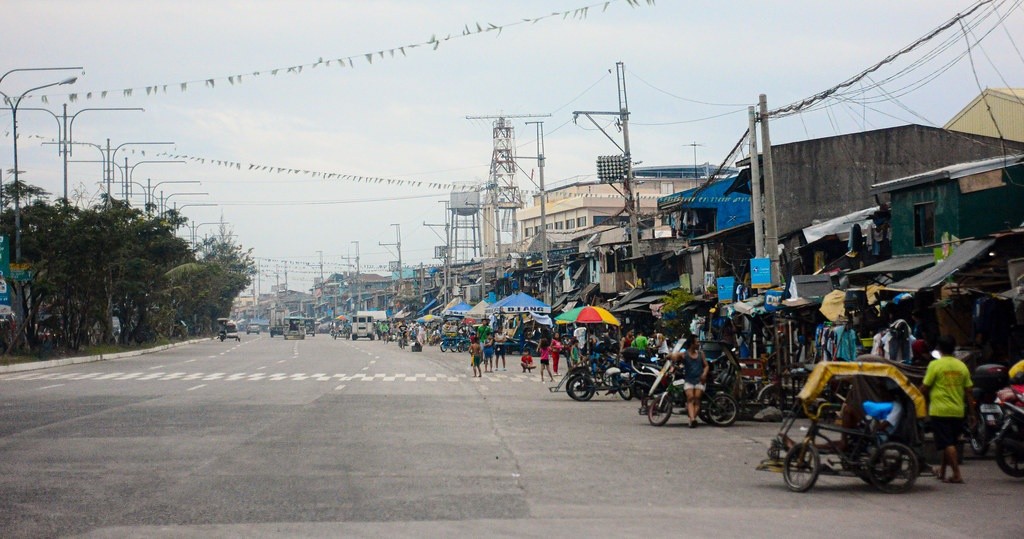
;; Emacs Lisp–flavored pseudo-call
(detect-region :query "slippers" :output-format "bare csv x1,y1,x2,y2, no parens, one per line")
944,477,964,483
935,469,944,478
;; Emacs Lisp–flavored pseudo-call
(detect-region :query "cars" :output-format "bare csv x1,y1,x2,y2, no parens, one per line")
246,322,260,334
221,321,241,342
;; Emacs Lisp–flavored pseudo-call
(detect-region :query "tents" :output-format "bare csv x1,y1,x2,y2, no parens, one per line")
440,291,551,319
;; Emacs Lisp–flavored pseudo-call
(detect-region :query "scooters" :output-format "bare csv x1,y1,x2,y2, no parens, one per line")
930,346,1007,461
438,321,850,427
330,328,351,340
216,318,229,342
986,364,1024,478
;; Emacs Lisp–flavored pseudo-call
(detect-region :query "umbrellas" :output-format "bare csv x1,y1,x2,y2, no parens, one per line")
555,305,620,356
335,315,346,326
415,314,443,323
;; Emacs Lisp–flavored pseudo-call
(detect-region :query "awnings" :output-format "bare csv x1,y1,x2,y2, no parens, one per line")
689,220,764,246
883,239,996,292
418,299,436,315
844,256,934,275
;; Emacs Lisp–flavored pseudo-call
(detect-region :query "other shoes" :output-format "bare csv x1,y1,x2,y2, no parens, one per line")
689,419,697,428
554,374,557,375
495,368,498,371
557,373,562,376
503,368,506,371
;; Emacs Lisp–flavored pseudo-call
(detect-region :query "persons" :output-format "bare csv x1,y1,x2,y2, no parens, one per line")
217,322,227,339
330,318,977,483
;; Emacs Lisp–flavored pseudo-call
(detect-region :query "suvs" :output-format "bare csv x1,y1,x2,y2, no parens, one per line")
268,316,316,341
351,315,377,341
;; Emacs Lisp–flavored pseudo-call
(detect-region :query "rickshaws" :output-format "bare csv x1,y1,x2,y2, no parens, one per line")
755,361,945,495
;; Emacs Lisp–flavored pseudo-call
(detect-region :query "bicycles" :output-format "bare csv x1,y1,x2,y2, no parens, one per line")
400,330,407,349
382,330,389,345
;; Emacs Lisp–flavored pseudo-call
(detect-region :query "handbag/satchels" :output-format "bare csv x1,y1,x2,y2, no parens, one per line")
637,350,649,362
553,348,562,352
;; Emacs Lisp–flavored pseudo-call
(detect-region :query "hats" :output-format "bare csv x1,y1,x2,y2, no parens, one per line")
911,340,933,354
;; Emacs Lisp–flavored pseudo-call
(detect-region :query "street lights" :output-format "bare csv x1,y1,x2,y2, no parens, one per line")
1,76,79,352
